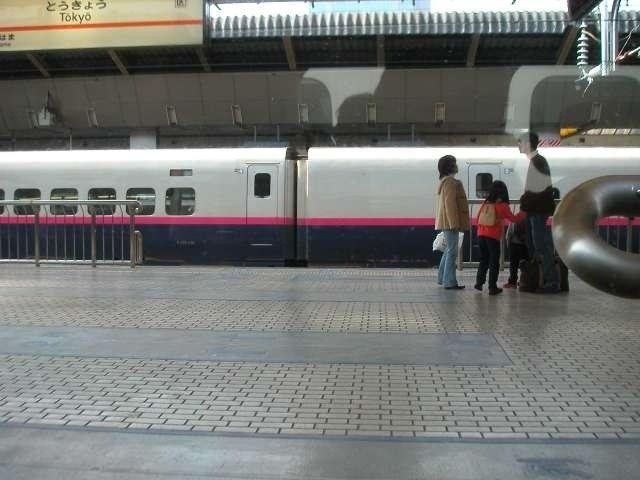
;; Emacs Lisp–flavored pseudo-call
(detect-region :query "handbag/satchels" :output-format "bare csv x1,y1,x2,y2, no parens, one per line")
519,257,569,292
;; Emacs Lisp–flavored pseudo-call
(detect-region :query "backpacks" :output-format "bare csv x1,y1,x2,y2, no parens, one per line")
478,202,500,227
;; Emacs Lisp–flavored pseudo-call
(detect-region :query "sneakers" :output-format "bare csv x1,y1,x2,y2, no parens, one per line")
474,283,482,290
536,287,546,293
489,287,502,295
438,281,465,290
502,283,516,288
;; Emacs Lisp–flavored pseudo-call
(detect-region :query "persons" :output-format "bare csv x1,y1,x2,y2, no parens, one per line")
473,179,525,295
518,131,559,294
435,154,470,290
502,187,571,293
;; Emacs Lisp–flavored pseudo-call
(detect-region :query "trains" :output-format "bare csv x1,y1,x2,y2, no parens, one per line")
0,146,640,268
